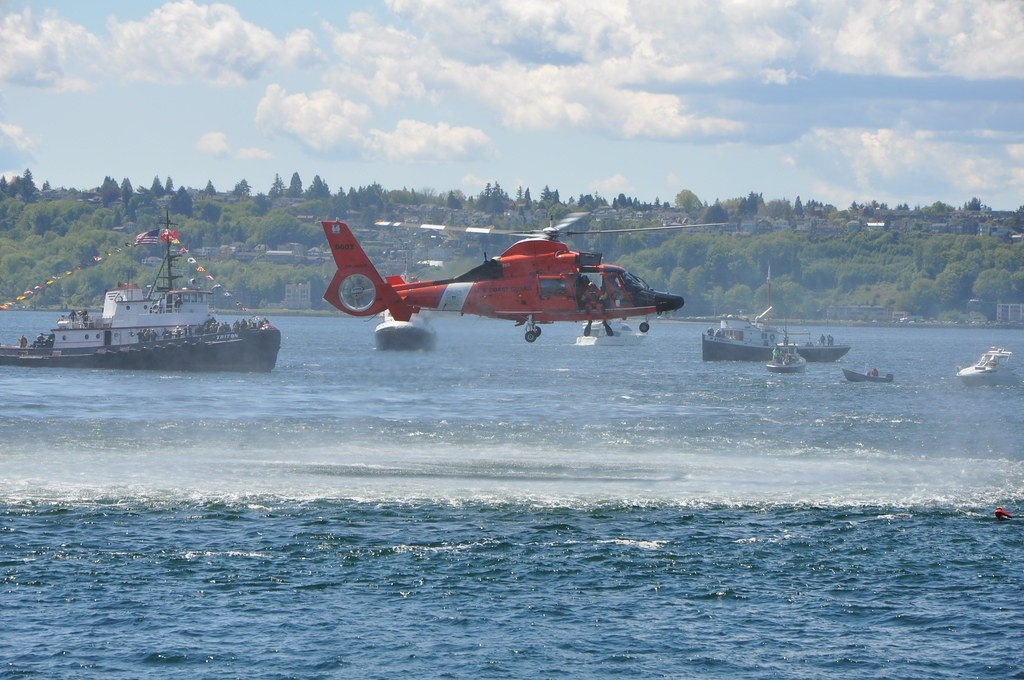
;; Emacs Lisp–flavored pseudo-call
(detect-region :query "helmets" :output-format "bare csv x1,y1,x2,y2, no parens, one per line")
588,282,594,287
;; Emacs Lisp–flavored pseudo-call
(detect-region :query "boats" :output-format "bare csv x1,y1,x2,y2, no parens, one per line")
995,505,1012,520
371,252,437,350
766,332,807,373
575,319,646,348
957,346,1019,385
717,306,785,346
841,367,894,382
0,209,283,376
702,276,851,363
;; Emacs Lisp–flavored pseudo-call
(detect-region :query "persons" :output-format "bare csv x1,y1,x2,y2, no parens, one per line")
151,296,165,313
580,280,614,337
771,346,780,361
36,333,45,343
136,315,271,343
865,363,879,377
69,308,77,322
820,333,834,347
707,327,722,342
18,335,28,349
175,297,183,314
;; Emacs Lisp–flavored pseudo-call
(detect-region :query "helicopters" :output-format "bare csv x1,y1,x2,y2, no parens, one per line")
320,210,728,342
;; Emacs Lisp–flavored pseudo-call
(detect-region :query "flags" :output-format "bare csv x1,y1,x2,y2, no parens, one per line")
162,228,178,243
0,242,132,310
136,229,159,244
172,238,260,321
